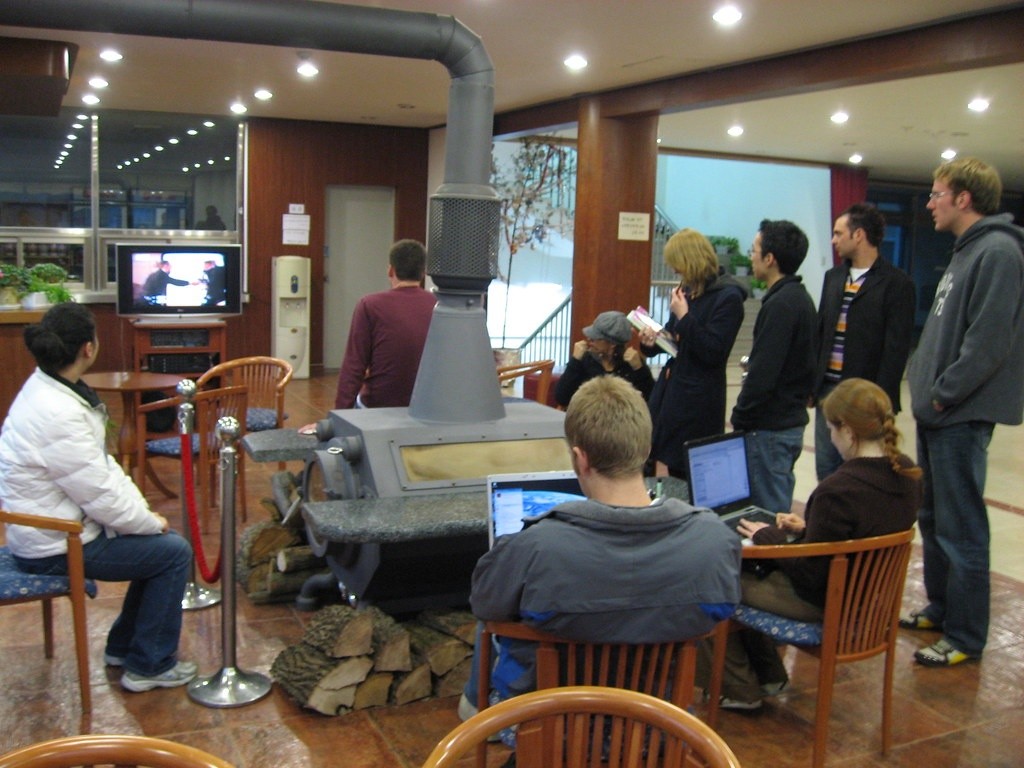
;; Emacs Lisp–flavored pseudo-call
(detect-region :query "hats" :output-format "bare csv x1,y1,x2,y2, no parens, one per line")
582,311,634,344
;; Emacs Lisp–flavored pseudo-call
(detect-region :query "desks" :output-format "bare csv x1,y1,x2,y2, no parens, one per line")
80,371,185,501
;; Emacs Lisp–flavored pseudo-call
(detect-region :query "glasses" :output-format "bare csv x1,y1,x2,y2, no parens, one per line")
929,189,954,200
746,248,765,259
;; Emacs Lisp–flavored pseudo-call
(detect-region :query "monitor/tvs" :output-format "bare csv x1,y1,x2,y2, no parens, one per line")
114,242,243,323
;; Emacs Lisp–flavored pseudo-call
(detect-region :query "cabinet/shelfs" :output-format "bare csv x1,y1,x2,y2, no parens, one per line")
126,317,230,441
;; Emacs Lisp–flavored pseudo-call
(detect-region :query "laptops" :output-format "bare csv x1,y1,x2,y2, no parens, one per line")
487,469,588,548
684,429,803,548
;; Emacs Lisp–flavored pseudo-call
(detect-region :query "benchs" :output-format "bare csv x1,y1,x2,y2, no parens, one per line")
239,426,319,462
301,472,693,553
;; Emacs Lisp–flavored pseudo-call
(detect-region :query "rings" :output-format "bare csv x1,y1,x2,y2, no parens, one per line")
650,336,653,341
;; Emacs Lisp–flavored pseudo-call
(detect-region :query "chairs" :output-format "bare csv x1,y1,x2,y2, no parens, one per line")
730,526,915,768
0,504,103,715
422,686,745,768
135,381,251,524
0,731,242,768
192,354,295,508
496,360,556,406
470,620,731,768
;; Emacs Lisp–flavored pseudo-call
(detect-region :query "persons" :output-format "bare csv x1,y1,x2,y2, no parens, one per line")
701,378,922,709
900,160,1024,666
457,374,743,742
140,260,226,306
298,239,438,434
556,311,653,412
646,228,750,481
730,220,824,515
0,301,199,692
814,202,915,483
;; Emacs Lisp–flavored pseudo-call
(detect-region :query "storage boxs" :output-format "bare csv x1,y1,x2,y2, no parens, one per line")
20,291,58,309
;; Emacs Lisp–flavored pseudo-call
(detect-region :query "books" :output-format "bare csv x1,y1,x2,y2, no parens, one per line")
626,305,678,358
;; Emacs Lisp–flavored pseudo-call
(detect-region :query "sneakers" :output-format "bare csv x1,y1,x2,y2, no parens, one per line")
120,660,198,693
764,680,791,696
898,608,939,630
701,686,762,709
914,639,981,668
103,630,135,666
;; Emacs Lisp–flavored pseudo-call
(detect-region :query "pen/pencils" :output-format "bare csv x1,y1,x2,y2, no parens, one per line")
656,477,662,496
675,278,687,296
